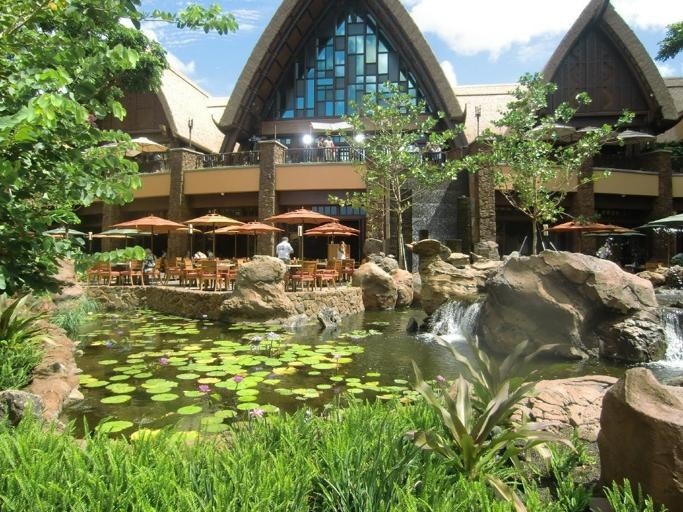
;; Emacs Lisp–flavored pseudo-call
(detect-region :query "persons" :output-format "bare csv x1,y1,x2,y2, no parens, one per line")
323,135,333,161
187,249,208,287
397,138,442,167
595,239,613,260
152,152,163,165
274,236,294,266
133,248,155,285
315,136,325,162
335,240,348,282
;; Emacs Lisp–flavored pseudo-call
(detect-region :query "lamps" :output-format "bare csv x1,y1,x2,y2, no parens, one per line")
187,116,194,149
273,111,279,139
475,104,482,138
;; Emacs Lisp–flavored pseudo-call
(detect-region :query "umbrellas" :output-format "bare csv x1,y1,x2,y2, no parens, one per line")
647,213,683,227
583,222,649,239
263,205,339,261
302,230,358,244
45,222,266,259
632,221,674,268
559,125,618,144
109,214,189,260
525,122,575,160
611,128,658,147
539,216,616,252
304,220,360,244
101,142,142,160
128,136,169,158
180,208,245,260
227,219,285,255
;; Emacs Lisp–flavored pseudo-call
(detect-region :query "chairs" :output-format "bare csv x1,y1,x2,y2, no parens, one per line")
87,256,355,292
